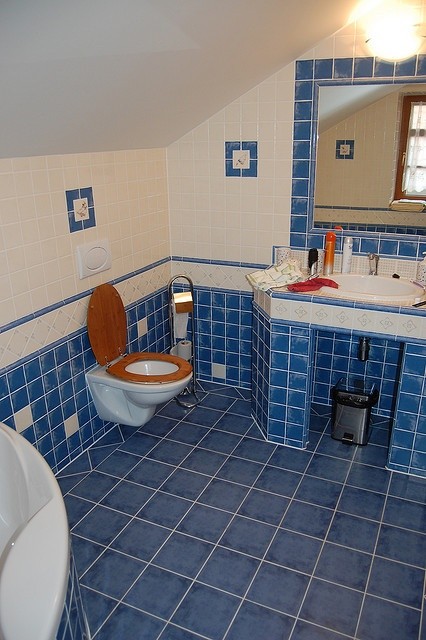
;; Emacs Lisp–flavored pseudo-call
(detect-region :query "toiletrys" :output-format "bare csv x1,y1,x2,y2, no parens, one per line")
341,236,353,274
308,248,318,276
334,226,343,230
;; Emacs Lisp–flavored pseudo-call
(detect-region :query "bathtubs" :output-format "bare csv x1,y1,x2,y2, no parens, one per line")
0,423,70,640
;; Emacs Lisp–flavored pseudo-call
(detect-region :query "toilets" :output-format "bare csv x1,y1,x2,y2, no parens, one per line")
84,285,193,427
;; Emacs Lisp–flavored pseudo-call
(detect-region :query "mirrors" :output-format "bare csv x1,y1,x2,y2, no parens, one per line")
290,55,426,260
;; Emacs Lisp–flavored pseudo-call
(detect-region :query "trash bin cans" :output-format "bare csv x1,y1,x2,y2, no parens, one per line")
331,377,378,445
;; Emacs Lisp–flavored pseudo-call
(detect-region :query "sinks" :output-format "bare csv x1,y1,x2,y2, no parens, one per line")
319,272,424,300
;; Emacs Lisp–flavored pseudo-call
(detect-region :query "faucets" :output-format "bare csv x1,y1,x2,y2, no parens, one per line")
369,253,379,275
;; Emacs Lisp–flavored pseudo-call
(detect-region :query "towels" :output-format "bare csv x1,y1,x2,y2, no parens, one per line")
246,259,303,292
390,198,426,213
288,277,339,293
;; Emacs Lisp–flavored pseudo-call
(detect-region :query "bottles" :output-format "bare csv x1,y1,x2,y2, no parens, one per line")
334,226,344,231
